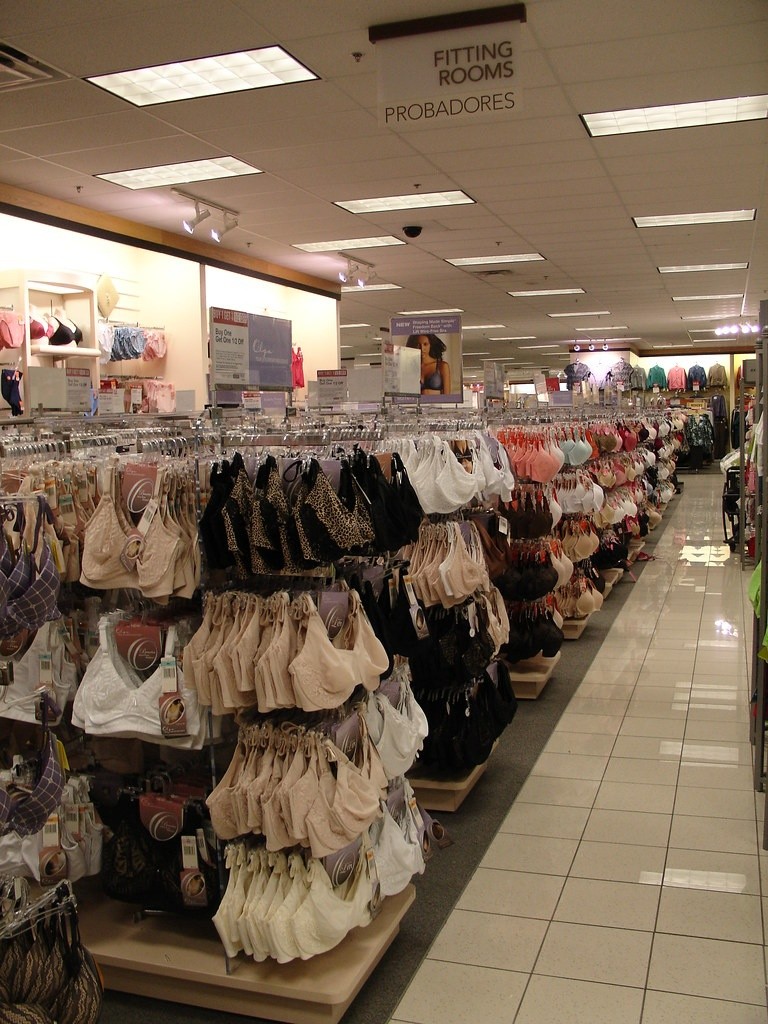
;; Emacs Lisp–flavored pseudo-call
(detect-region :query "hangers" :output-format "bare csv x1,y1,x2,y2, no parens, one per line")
0,305,168,386
1,390,725,939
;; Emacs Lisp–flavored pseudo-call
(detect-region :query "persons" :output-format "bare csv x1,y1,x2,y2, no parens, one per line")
290,343,305,389
47,308,82,348
406,334,451,395
29,304,49,345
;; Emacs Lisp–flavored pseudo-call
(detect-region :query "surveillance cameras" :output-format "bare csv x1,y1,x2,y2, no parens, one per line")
402,226,422,238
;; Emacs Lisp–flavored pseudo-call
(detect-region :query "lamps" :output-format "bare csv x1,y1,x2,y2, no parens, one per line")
338,252,377,287
171,188,239,242
573,339,608,351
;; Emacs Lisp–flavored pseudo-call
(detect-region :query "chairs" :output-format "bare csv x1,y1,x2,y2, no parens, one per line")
722,466,747,544
